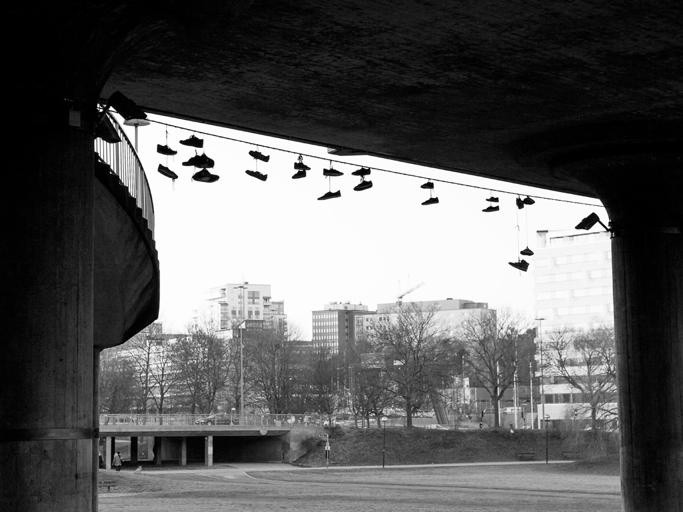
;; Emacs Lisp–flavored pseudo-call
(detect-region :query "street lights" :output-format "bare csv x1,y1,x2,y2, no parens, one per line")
236,319,247,424
535,318,544,430
544,414,550,465
381,416,388,468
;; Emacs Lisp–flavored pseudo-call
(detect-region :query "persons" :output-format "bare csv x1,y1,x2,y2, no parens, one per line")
110,451,125,471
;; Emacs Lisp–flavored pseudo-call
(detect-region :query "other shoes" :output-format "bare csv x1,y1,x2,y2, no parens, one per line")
245,170,268,181
323,168,344,176
482,206,499,212
292,170,306,179
294,162,311,170
354,180,372,191
157,143,177,155
516,198,524,209
192,168,219,183
352,166,370,175
249,151,270,162
486,197,498,202
182,153,214,168
158,164,178,179
509,259,529,272
318,190,341,200
422,197,439,205
180,135,204,148
520,247,534,256
524,198,535,204
421,182,433,188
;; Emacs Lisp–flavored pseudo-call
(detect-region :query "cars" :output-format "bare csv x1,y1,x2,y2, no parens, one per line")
195,413,239,425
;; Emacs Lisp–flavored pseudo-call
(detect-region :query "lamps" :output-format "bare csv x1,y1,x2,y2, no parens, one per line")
572,212,612,233
